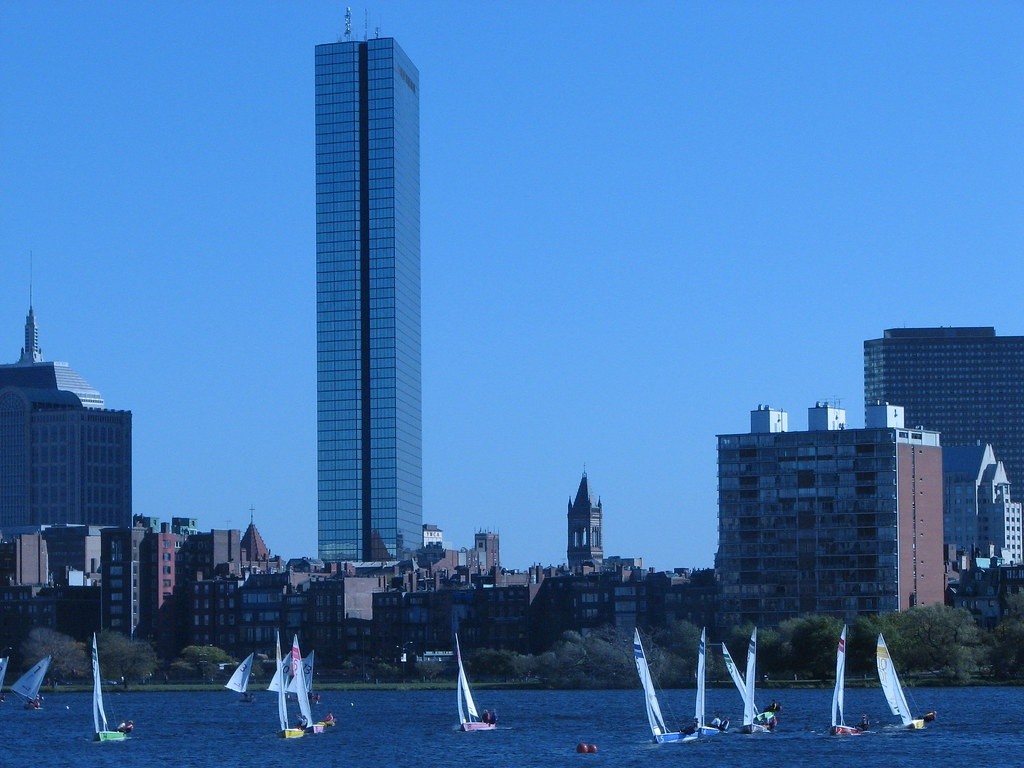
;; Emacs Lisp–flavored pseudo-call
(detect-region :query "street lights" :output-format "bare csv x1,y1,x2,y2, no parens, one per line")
202,643,212,686
396,641,413,672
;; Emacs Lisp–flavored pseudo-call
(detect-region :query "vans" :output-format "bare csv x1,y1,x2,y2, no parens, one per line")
218,663,255,681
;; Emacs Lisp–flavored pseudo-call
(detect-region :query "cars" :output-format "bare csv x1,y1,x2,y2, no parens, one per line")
54,678,72,685
101,679,117,685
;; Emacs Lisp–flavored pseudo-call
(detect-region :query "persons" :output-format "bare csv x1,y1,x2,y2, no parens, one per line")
712,712,721,727
857,714,869,731
919,711,937,722
481,708,498,724
115,720,134,734
326,711,333,721
771,699,777,709
297,716,308,730
28,699,33,704
308,690,312,698
315,693,320,700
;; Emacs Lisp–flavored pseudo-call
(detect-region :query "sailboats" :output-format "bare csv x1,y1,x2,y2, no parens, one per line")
292,635,327,736
740,626,767,734
876,632,925,732
694,626,722,736
267,651,294,704
8,654,53,709
286,650,319,705
631,627,699,744
276,631,304,739
222,652,256,703
454,632,497,733
90,632,126,742
720,642,778,730
0,657,9,704
829,624,860,736
